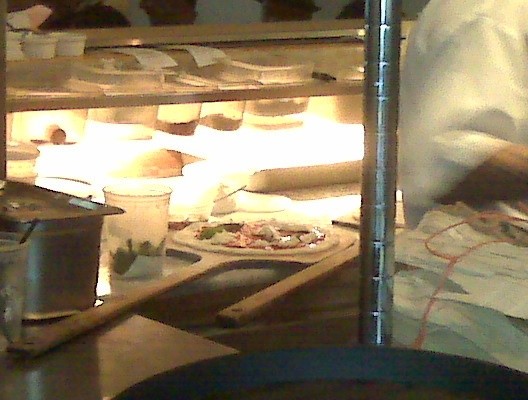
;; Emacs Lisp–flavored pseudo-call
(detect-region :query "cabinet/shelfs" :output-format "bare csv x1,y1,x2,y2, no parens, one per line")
0,20,414,400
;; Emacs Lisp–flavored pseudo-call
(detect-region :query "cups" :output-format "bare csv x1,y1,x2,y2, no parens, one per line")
103,184,174,289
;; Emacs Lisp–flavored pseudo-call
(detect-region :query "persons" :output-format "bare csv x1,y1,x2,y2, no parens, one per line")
396,0,528,229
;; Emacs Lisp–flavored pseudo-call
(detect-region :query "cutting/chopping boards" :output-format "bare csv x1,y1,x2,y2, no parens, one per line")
217,202,406,329
5,210,357,361
